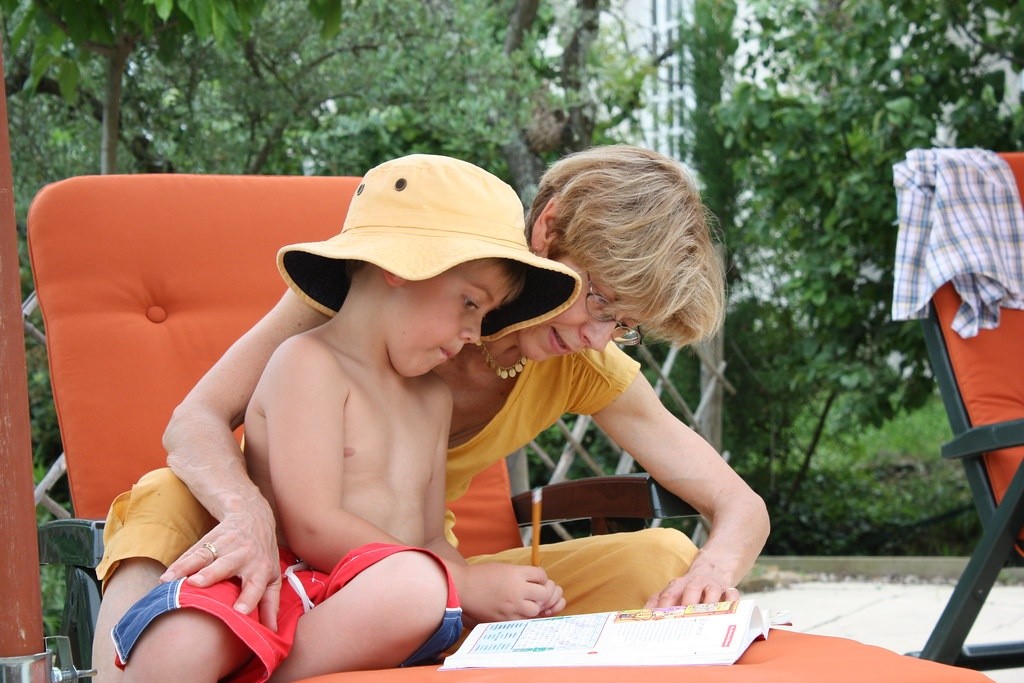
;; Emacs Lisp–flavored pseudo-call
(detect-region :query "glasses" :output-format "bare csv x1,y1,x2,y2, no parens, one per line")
585,270,643,353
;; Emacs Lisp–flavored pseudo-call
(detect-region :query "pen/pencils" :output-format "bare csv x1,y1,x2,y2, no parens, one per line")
531,485,543,567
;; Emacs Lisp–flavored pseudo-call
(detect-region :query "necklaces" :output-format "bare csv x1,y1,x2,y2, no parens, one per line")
475,339,530,380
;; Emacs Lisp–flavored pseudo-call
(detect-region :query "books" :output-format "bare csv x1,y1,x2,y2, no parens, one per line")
438,603,792,669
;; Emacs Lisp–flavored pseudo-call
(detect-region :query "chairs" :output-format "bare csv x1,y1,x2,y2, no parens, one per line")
889,149,1023,674
25,173,996,683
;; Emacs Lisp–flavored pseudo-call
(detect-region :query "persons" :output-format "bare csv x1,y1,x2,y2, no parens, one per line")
89,143,770,683
241,151,583,683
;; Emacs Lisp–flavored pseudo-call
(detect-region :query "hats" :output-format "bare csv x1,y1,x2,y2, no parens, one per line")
275,153,579,342
